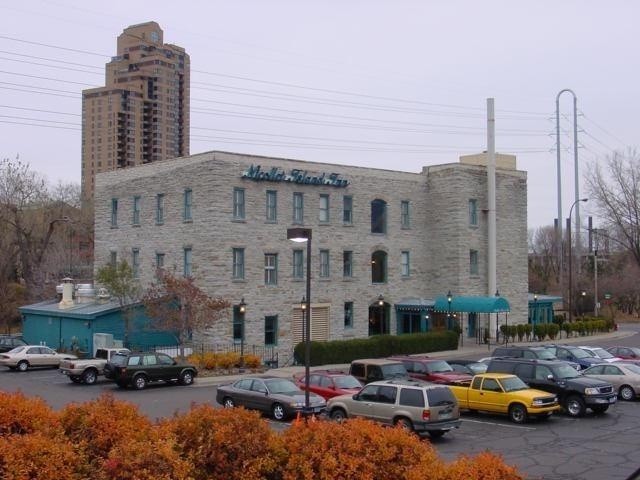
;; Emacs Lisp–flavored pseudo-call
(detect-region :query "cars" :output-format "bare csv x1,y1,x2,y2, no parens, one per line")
0,345,79,372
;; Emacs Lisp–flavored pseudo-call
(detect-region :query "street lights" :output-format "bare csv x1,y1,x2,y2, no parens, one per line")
445,288,454,334
494,289,501,345
567,197,589,338
377,293,385,337
299,294,307,366
533,288,538,341
424,314,430,332
453,313,457,332
581,287,586,338
284,224,314,428
236,297,248,375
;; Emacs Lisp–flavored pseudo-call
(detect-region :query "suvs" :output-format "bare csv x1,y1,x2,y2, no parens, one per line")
103,350,199,391
0,333,31,353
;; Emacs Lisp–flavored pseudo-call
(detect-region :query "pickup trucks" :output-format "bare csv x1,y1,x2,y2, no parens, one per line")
59,346,133,384
